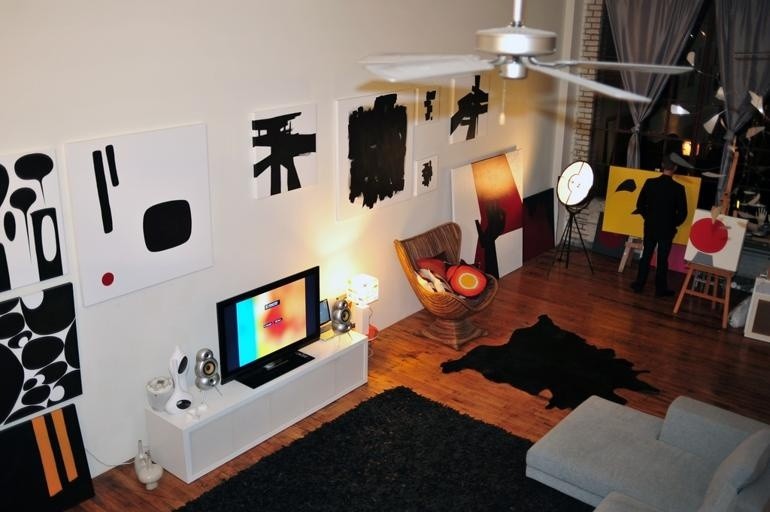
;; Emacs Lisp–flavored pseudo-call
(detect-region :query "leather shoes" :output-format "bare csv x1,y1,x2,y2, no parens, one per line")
630,282,645,294
654,289,675,298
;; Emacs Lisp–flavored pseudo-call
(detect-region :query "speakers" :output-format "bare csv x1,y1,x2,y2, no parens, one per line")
332,301,352,336
194,347,219,390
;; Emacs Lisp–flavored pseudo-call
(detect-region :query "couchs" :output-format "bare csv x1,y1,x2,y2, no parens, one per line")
523,391,770,510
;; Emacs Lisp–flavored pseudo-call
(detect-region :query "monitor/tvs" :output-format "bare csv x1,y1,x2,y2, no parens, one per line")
216,264,321,389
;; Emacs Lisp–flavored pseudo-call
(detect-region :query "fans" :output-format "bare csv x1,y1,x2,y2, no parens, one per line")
358,0,694,103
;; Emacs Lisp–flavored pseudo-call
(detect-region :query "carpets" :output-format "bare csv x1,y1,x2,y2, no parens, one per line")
172,386,593,512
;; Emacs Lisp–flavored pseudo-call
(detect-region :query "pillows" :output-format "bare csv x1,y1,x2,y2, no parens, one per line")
413,249,488,300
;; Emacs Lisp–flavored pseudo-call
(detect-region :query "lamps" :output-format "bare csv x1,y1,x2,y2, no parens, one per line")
346,274,379,359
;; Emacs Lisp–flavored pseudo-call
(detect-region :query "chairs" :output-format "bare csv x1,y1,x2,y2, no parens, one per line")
394,221,500,350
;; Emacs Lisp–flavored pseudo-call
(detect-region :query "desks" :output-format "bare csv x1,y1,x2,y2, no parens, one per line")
146,325,370,484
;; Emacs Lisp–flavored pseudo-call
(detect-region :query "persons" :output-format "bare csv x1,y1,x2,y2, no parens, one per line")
630,154,688,299
